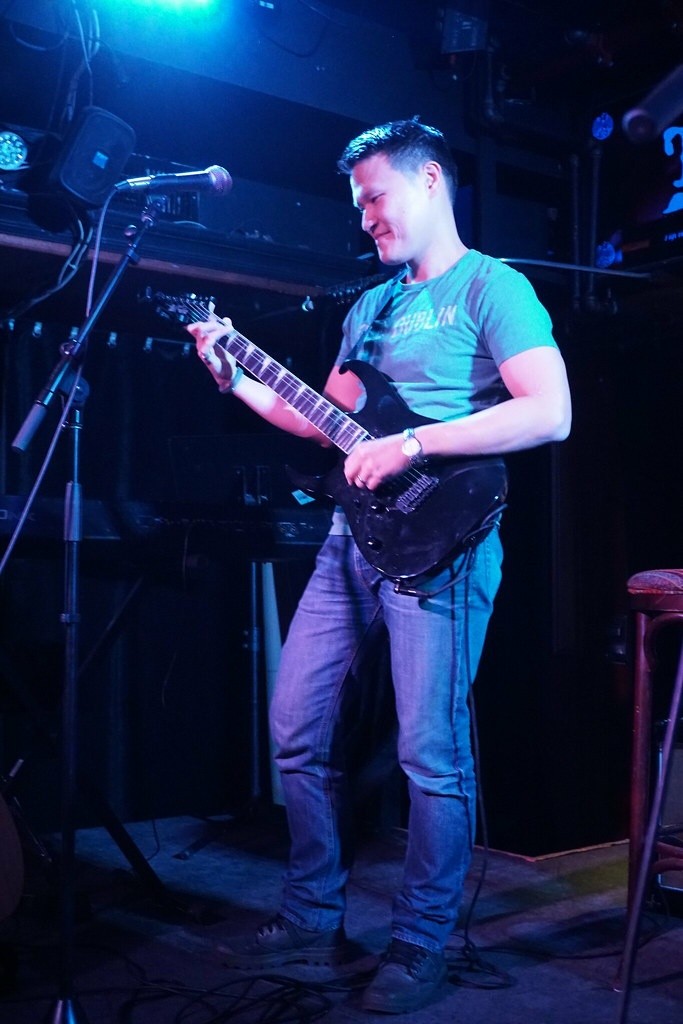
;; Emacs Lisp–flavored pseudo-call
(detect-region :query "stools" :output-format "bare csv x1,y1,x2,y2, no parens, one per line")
615,568,683,993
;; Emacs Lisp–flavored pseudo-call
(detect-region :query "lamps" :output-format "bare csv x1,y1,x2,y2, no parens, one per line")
592,108,658,146
0,123,31,169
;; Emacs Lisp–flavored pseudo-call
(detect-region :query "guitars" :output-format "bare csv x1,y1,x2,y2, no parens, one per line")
143,283,509,586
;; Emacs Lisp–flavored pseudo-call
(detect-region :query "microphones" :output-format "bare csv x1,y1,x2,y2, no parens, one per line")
114,165,233,195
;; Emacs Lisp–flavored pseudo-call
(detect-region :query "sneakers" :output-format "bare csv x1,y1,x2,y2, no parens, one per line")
361,938,449,1015
213,913,352,971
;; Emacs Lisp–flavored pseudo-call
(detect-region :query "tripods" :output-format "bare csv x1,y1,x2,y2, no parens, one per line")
10,195,203,1024
